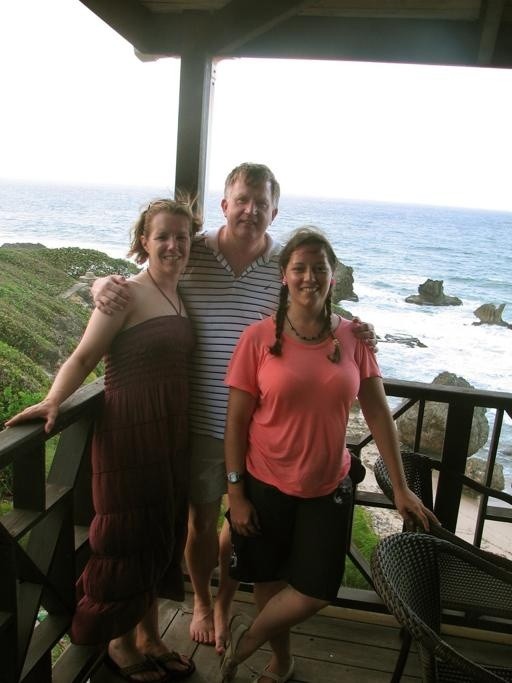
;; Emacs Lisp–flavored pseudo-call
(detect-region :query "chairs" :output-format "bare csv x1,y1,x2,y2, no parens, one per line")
369,452,512,568
370,532,512,683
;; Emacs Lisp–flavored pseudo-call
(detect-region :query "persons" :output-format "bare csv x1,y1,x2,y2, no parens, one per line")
89,162,378,654
214,225,443,682
4,186,197,683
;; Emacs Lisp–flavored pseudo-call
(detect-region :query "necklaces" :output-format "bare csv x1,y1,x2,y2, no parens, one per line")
286,310,327,341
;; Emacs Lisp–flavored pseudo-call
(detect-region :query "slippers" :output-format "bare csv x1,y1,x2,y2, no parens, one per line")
153,648,197,676
104,648,170,683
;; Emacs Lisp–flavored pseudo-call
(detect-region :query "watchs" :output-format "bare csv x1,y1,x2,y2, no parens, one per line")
225,471,244,484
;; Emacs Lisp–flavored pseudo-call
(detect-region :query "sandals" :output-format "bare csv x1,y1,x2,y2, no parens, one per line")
256,657,296,683
220,614,253,682
